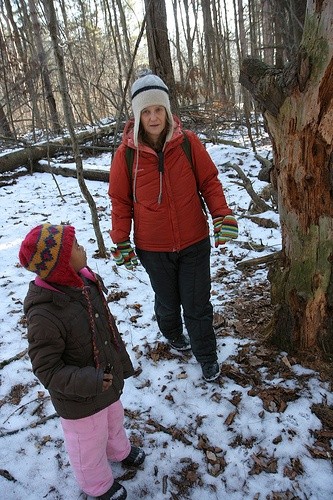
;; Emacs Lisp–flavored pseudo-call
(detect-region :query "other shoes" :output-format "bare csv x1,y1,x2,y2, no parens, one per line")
167,333,192,351
200,360,221,382
121,445,146,467
98,480,127,500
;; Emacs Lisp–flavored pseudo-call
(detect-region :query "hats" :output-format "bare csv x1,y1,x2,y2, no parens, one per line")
18,222,123,368
130,68,175,204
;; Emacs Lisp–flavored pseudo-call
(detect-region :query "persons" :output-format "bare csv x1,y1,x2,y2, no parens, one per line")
108,63,239,382
18,223,146,500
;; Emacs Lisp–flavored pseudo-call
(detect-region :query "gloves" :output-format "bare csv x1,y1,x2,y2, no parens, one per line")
212,215,239,247
110,238,139,270
